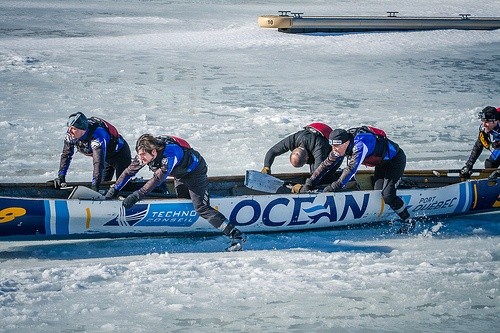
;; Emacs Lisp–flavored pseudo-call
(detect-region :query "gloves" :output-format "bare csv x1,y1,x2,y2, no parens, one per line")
262,167,271,175
121,194,139,209
488,171,499,179
460,166,472,178
323,182,340,193
106,187,119,200
47,175,66,188
299,178,316,194
292,184,303,194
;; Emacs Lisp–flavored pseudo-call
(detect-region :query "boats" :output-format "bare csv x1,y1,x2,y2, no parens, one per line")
0,166,500,240
258,10,500,30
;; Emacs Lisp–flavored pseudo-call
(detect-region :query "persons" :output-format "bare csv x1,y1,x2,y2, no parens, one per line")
261,123,360,192
106,134,243,237
460,106,500,181
47,112,131,192
300,126,410,221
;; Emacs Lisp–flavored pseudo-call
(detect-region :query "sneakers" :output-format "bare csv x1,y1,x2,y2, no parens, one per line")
399,219,415,233
225,231,247,252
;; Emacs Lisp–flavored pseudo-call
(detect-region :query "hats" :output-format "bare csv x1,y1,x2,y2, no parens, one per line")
478,106,498,121
329,129,350,146
66,112,88,131
